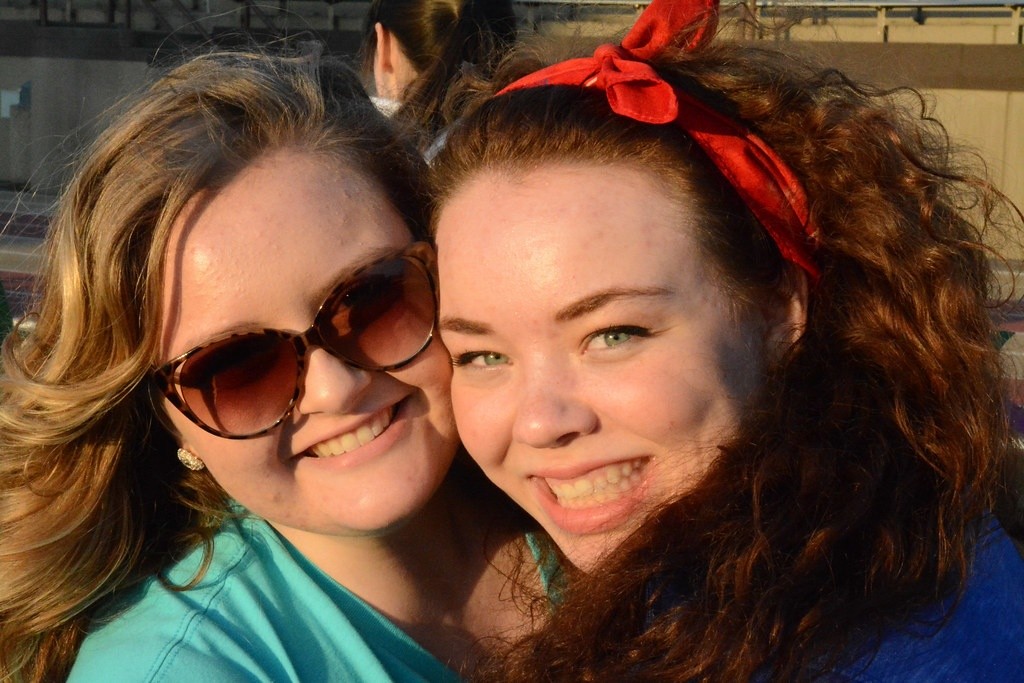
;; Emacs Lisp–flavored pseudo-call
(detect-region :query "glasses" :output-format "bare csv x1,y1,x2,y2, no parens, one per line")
140,238,439,441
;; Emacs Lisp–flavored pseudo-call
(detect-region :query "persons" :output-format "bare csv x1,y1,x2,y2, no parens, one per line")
364,0,518,156
406,1,1024,682
0,1,593,682
